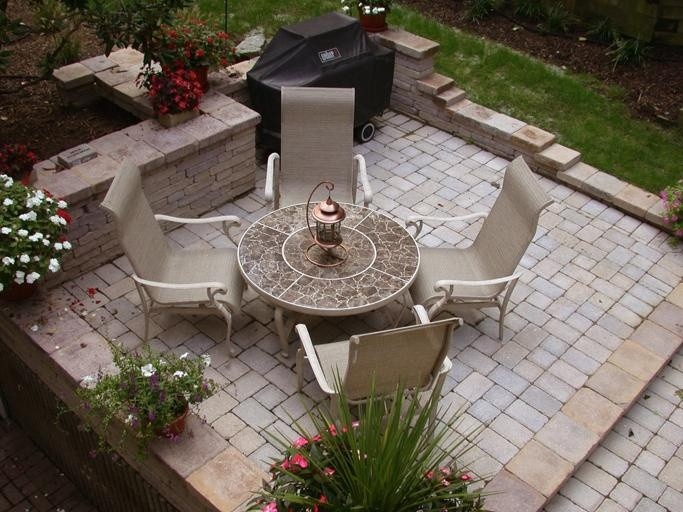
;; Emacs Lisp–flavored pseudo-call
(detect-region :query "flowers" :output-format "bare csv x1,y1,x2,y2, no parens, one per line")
54,338,222,468
134,3,240,118
244,363,509,511
1,144,74,293
341,0,392,15
658,180,683,249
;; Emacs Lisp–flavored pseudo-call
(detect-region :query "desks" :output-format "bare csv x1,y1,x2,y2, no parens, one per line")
237,199,421,357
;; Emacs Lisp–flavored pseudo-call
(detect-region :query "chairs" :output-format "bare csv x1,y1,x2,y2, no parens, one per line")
401,155,554,342
101,158,248,358
262,87,373,211
295,305,463,422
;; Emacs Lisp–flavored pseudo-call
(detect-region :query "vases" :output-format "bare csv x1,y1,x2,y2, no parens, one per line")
154,396,189,438
359,13,388,33
4,281,40,301
